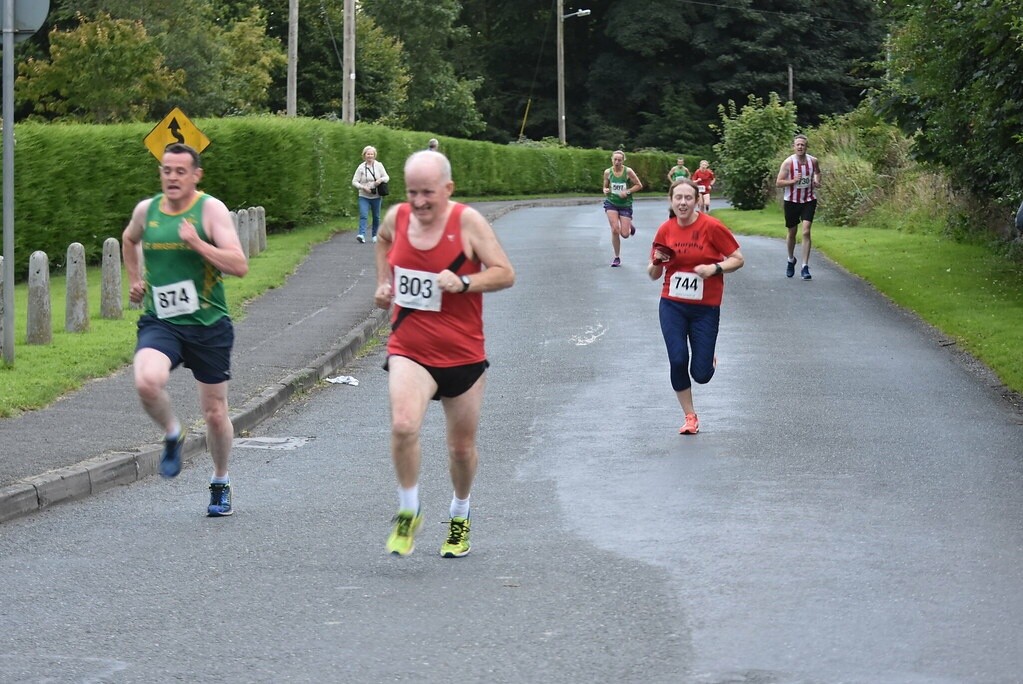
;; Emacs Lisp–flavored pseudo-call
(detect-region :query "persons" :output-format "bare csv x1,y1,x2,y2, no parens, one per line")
646,178,744,434
667,156,691,184
122,143,250,513
373,149,513,560
775,134,821,281
601,150,643,267
427,139,439,152
352,146,389,245
691,160,716,213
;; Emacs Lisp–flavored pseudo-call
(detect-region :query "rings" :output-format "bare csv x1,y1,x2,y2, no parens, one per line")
447,283,452,287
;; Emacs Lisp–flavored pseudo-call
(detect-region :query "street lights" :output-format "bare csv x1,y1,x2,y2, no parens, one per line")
557,11,592,144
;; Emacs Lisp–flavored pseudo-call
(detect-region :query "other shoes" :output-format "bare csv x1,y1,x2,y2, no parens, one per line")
706,205,709,211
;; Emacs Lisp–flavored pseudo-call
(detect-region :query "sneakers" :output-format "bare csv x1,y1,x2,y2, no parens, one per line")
679,413,699,434
629,223,636,236
387,501,424,557
611,256,620,267
786,257,798,276
356,235,366,243
801,264,812,281
157,422,187,479
372,236,378,244
442,511,472,557
206,476,233,516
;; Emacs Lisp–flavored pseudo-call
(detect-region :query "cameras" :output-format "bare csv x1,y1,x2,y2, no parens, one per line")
370,188,377,194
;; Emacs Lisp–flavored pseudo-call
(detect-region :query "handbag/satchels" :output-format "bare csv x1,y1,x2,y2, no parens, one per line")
377,182,390,196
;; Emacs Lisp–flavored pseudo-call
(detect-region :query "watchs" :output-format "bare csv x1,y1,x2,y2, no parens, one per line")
625,189,631,196
714,262,723,274
459,274,470,293
